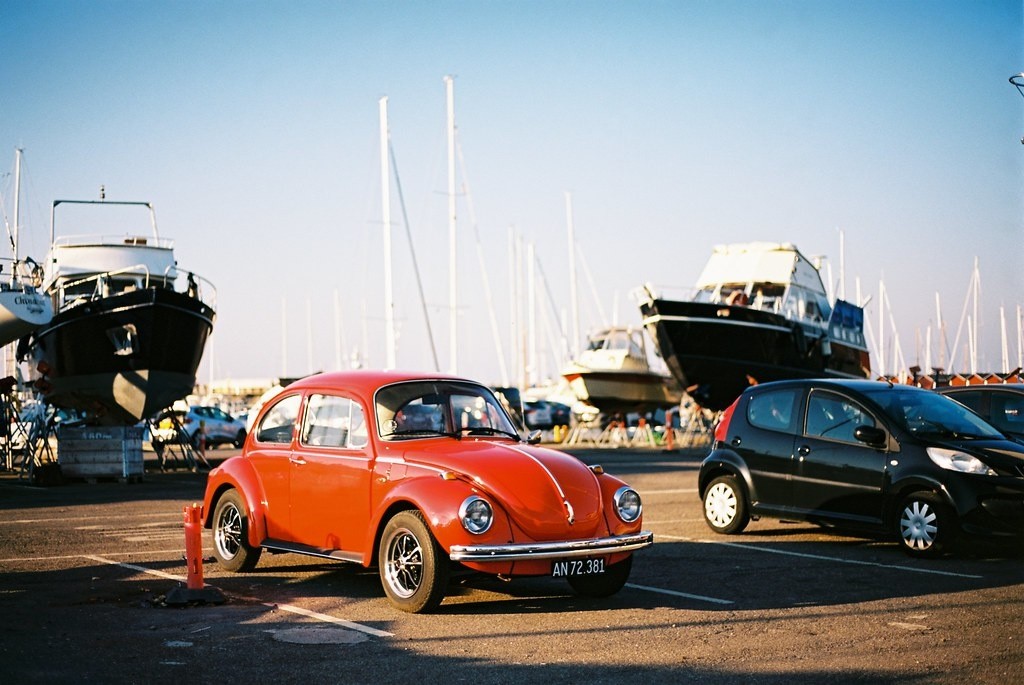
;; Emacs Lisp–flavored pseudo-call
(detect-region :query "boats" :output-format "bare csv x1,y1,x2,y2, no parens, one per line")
562,330,683,413
637,240,873,412
21,193,218,428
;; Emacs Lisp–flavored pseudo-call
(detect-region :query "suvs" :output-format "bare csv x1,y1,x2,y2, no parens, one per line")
151,406,246,449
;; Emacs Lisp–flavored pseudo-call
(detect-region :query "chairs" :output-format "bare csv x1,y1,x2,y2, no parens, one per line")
816,406,858,440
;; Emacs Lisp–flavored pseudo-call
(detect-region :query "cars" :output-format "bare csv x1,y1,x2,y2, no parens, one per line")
695,375,1024,553
198,369,654,614
932,383,1024,444
